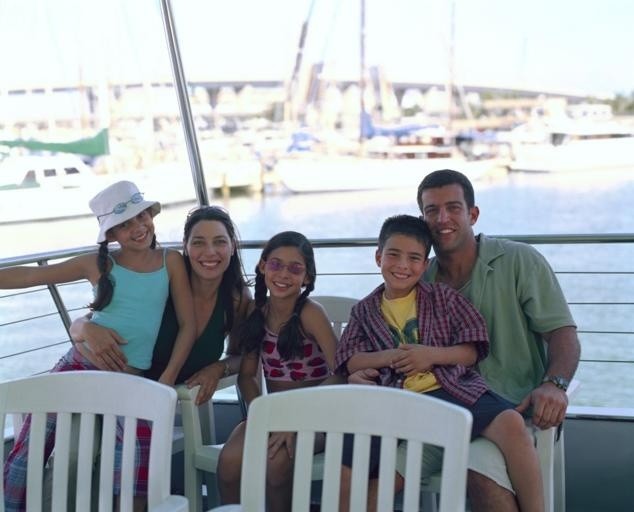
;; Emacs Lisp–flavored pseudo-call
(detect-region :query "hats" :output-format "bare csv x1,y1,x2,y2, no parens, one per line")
90,180,162,245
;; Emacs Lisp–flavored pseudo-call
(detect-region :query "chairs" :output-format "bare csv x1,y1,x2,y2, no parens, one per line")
240,382,475,512
421,398,567,512
160,384,221,504
0,369,178,512
176,294,361,512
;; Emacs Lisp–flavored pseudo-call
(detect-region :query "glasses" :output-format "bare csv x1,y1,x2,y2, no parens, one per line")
263,258,307,275
98,191,144,219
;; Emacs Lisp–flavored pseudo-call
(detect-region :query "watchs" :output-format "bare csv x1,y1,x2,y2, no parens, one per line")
541,375,568,392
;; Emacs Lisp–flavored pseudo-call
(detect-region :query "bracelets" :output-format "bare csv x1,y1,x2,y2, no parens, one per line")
220,359,233,377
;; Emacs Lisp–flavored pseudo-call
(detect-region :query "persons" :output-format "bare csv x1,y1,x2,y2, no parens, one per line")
1,179,197,512
67,206,253,512
334,213,547,512
346,169,581,511
217,231,351,512
